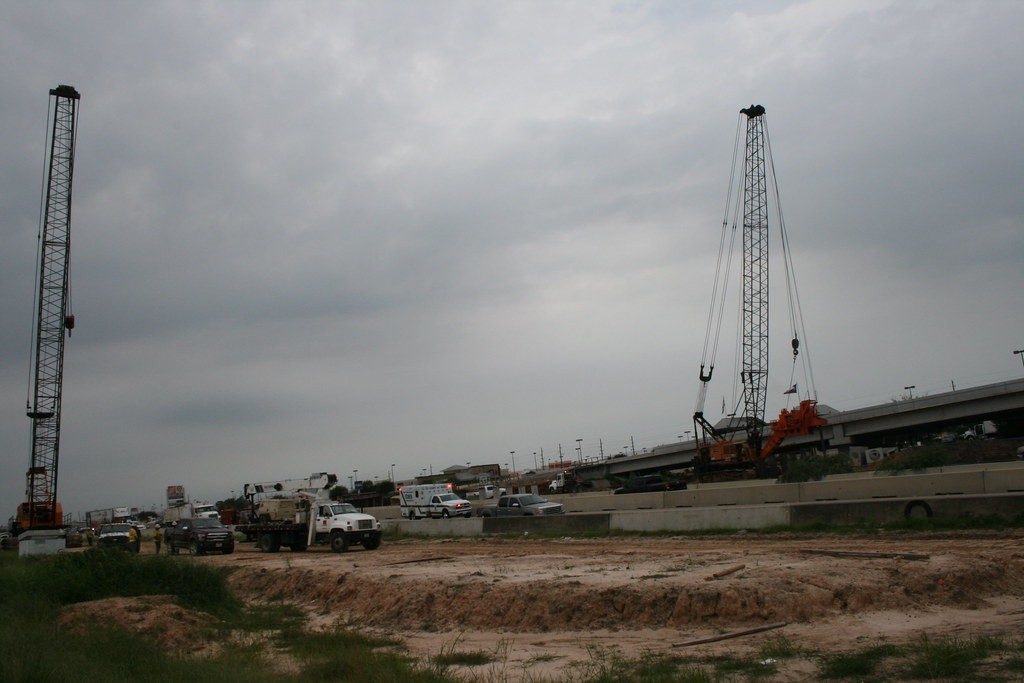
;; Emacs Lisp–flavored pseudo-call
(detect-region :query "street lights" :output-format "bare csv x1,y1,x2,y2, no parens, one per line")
574,439,583,466
352,470,358,494
349,477,353,492
533,452,538,472
684,431,691,440
678,436,683,442
391,463,395,490
1013,350,1024,366
510,451,515,473
904,386,915,400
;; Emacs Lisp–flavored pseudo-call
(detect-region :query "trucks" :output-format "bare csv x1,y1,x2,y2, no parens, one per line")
162,504,222,528
478,485,506,500
234,474,381,552
563,466,611,493
399,484,472,520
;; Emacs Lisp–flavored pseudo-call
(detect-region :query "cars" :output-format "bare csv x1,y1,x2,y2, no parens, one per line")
549,480,557,493
80,517,163,554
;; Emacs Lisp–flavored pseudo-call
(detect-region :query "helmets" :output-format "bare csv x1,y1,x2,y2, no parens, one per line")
155,524,161,528
131,523,136,526
87,527,91,529
172,521,177,525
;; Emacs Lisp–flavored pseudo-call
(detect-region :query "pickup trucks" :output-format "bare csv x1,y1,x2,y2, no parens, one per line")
477,494,565,518
613,475,687,495
164,518,235,557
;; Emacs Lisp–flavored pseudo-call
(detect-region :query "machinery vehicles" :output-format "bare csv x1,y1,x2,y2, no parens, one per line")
9,85,83,549
690,104,827,482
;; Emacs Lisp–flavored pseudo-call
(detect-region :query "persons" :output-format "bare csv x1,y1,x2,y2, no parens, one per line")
86,527,93,546
153,524,162,553
128,524,140,553
